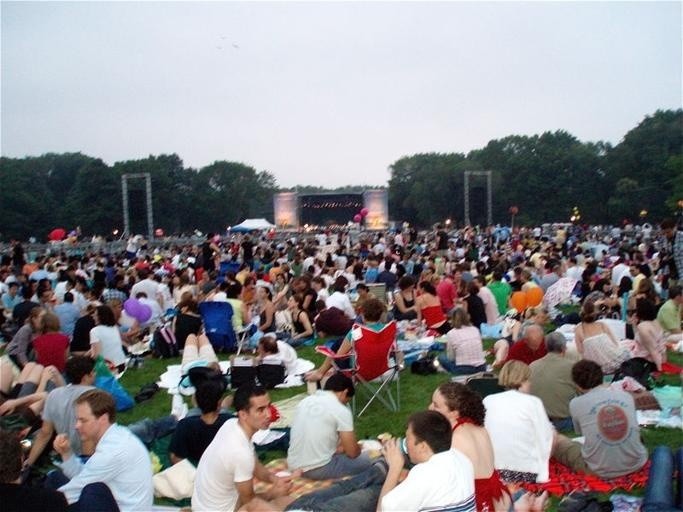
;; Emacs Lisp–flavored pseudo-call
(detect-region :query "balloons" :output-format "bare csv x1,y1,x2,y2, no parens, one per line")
354,208,368,222
507,206,517,214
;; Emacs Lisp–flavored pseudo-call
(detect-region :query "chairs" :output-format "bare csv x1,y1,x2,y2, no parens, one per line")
198,300,256,357
366,283,394,311
313,320,400,417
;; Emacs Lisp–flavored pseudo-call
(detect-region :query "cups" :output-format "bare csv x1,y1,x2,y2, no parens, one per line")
275,472,292,491
384,437,408,456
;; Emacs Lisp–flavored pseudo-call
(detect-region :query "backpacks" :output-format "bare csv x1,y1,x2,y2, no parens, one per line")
257,364,286,389
188,366,227,391
153,326,179,359
230,365,255,390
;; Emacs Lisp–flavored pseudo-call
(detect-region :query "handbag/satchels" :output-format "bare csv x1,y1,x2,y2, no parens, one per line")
560,493,614,512
120,326,142,344
612,357,656,390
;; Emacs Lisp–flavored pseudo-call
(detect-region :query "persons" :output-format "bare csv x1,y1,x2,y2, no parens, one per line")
552,360,648,481
482,359,557,484
190,385,303,512
427,382,513,512
21,356,188,473
375,411,476,512
634,445,682,512
179,455,388,512
51,389,154,512
168,369,239,468
286,374,370,480
513,490,548,512
0,426,71,512
0,218,682,441
